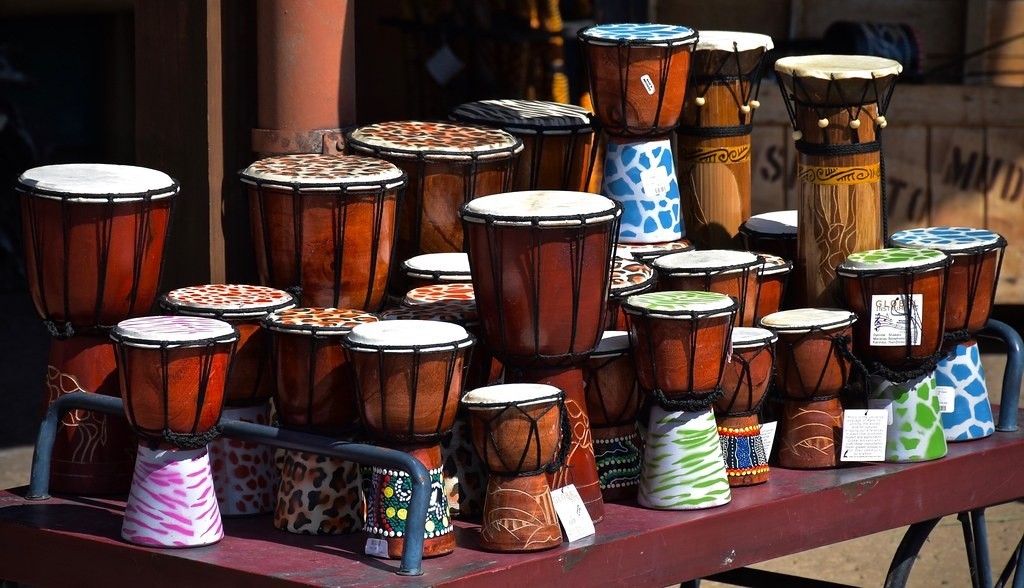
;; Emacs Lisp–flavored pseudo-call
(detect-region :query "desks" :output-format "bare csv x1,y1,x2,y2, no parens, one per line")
0,319,1024,588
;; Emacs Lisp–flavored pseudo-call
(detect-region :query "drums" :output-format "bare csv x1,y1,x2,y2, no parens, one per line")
773,55,903,308
739,210,797,308
835,247,955,464
576,23,699,244
377,252,793,519
339,320,477,558
345,120,524,312
259,307,380,535
678,29,775,250
16,164,181,497
108,316,240,547
714,326,781,487
759,308,860,470
887,227,1009,442
457,191,623,526
582,331,643,503
461,384,568,551
450,97,597,191
235,154,405,315
622,291,741,511
159,285,299,515
